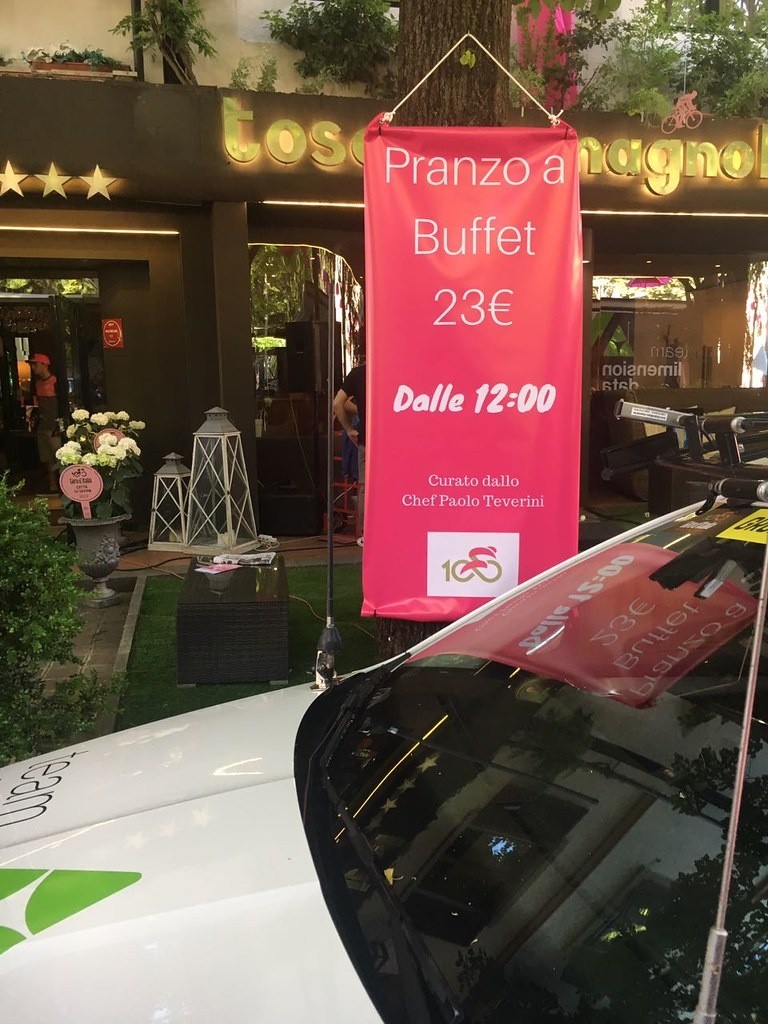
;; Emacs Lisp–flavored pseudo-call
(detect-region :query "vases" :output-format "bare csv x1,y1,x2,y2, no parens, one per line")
56,513,129,609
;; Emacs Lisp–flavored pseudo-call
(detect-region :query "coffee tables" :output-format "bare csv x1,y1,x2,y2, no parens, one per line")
175,552,293,684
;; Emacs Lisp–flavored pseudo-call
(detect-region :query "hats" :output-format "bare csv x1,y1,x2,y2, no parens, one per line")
25,353,50,365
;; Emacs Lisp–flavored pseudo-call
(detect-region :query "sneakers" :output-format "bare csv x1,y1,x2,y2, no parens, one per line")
36,489,61,497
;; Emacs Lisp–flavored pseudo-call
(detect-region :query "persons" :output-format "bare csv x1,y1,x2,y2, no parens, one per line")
25,354,68,497
333,363,365,547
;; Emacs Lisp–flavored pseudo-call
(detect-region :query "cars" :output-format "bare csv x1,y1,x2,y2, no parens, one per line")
2,468,766,1024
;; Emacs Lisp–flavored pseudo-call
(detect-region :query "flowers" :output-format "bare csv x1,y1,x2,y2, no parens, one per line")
55,406,145,519
